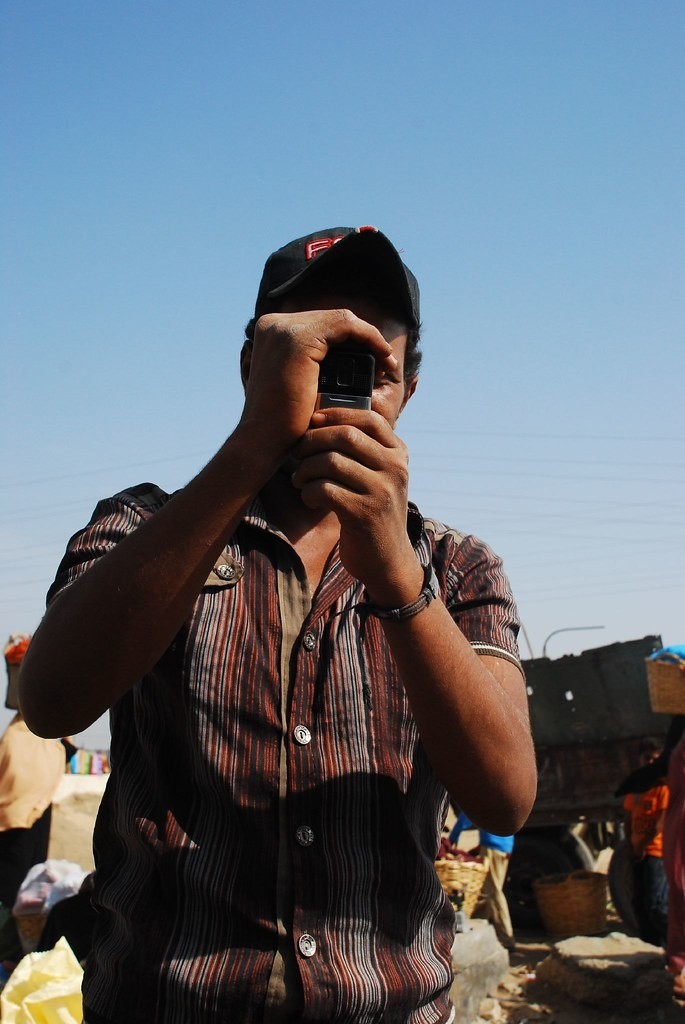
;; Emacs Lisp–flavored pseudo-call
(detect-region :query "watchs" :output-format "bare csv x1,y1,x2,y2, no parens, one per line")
362,563,440,620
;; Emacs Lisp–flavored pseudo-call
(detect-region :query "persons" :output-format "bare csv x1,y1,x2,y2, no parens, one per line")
11,224,536,1024
0,634,685,1024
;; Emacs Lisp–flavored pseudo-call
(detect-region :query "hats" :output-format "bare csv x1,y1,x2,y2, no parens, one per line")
255,225,420,328
4,633,32,667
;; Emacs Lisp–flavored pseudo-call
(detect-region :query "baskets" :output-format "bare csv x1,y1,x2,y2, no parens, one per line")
644,652,685,714
532,869,608,938
434,855,489,920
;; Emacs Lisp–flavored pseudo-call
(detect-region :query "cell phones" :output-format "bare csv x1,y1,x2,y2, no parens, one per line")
313,351,375,414
